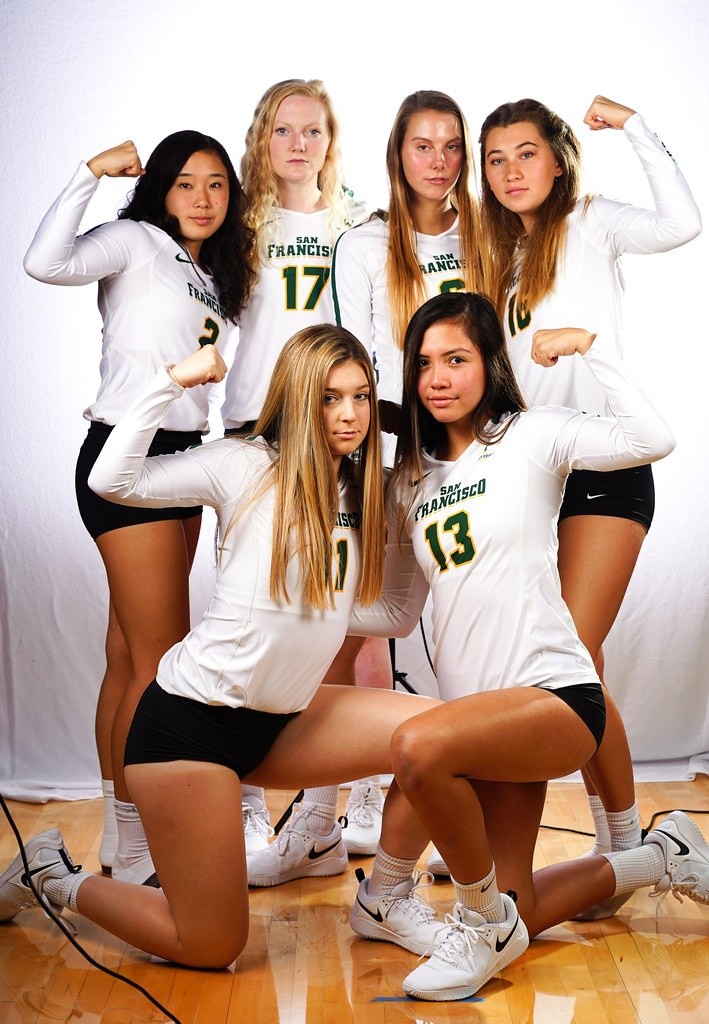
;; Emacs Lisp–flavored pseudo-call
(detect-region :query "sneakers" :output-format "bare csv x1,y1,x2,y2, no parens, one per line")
403,892,529,1000
644,811,709,906
428,851,450,876
350,878,451,957
245,820,348,885
243,796,269,854
0,830,77,922
341,783,385,854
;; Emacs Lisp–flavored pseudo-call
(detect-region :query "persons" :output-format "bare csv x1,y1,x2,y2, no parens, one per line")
246,88,486,890
23,129,248,893
348,291,708,1003
477,98,702,924
3,321,454,969
222,81,391,863
